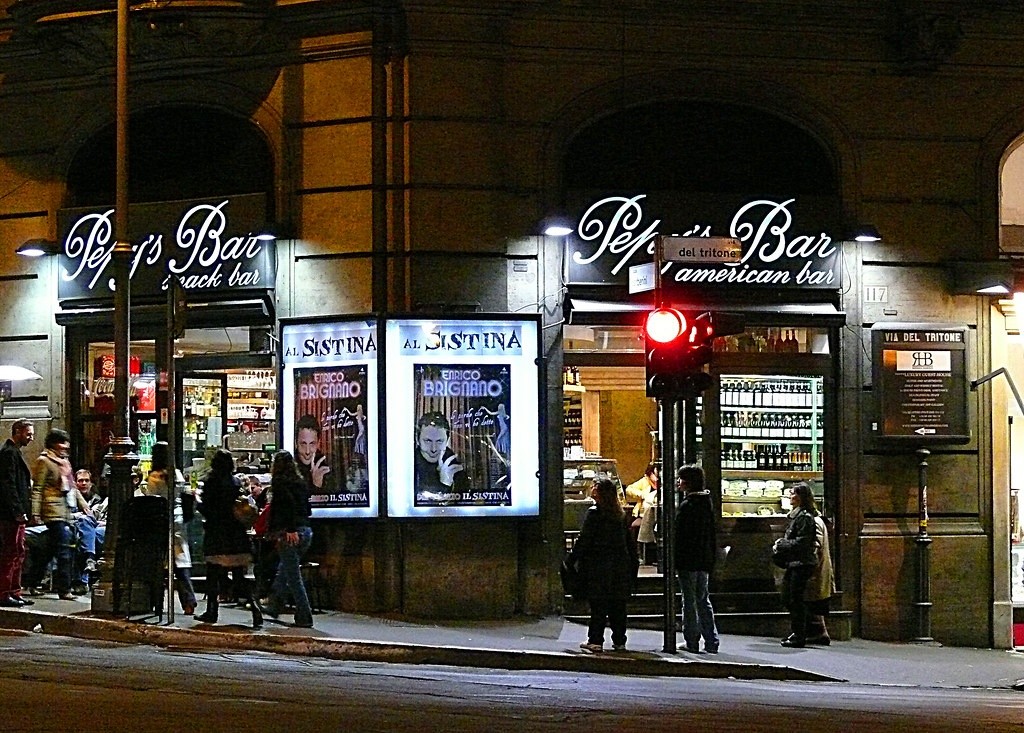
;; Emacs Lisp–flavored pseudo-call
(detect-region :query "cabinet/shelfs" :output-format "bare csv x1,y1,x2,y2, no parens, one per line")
696,374,823,518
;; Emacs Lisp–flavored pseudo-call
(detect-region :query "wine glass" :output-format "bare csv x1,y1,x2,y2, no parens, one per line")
228,399,276,419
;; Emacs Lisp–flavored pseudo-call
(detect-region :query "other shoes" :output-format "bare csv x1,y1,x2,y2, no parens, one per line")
183,599,196,614
781,635,804,648
0,597,24,607
806,635,831,645
611,645,625,651
580,644,603,654
194,615,217,623
293,620,313,626
60,593,77,599
680,645,699,654
12,596,34,605
702,648,718,655
261,606,278,618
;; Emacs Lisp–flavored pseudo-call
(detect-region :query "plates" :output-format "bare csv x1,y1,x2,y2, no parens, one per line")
586,456,603,459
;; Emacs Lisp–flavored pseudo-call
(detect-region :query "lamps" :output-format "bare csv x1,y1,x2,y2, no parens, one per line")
248,224,292,242
853,224,882,243
16,238,69,261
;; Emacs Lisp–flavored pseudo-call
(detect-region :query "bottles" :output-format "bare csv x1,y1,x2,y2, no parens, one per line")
183,386,220,447
714,328,800,354
562,364,582,457
719,381,822,470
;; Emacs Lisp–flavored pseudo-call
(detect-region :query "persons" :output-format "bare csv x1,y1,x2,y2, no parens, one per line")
63,469,109,592
295,414,335,497
415,411,471,494
32,427,88,600
234,472,272,532
258,450,313,627
671,465,719,654
624,461,659,564
771,481,833,647
0,417,35,607
194,450,263,625
140,441,197,615
563,476,632,654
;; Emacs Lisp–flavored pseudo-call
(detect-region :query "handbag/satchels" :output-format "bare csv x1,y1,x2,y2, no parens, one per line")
232,477,259,529
255,504,271,536
